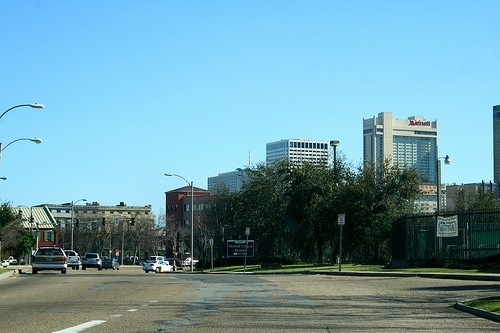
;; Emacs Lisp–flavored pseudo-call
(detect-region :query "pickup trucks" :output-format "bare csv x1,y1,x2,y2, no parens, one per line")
3,256,23,264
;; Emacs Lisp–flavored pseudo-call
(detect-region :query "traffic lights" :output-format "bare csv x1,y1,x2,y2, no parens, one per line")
76,219,80,227
30,218,34,223
19,210,22,218
131,218,135,226
102,217,105,225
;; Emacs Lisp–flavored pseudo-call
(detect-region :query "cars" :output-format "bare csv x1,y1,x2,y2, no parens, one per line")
143,260,174,273
31,247,67,274
64,250,80,270
83,253,102,271
102,257,119,270
129,256,199,267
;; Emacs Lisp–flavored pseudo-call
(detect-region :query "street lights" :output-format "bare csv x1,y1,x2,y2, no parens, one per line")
436,154,450,215
165,173,193,273
71,198,88,249
